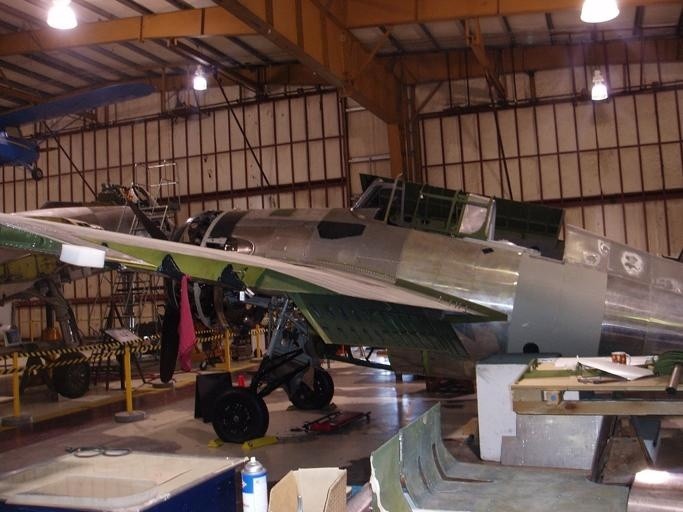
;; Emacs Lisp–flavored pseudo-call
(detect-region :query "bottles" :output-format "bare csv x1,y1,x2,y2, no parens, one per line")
240,456,268,512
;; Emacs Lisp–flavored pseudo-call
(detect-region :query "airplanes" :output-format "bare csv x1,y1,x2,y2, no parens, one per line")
0,79,156,182
0,171,683,444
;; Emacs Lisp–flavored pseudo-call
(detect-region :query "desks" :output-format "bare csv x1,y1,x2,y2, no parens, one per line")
509,355,683,482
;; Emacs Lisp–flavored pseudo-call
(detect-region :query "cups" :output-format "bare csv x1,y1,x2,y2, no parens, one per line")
610,351,632,366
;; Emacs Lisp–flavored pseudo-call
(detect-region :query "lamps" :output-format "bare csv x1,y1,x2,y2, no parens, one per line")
581,0,621,25
46,0,79,31
591,68,609,101
191,63,208,92
